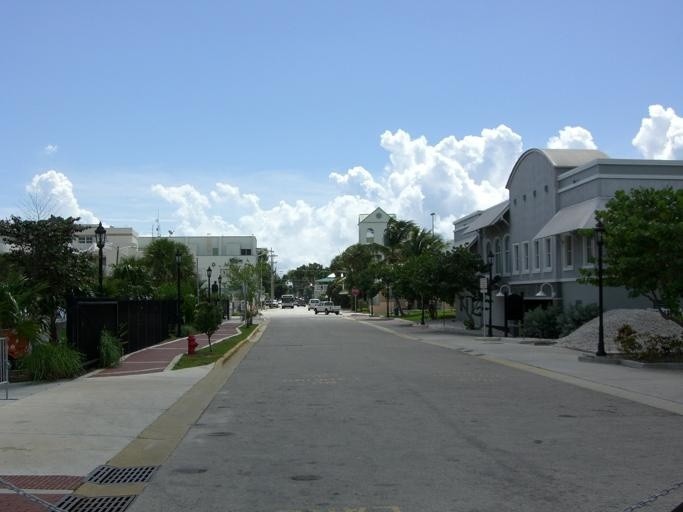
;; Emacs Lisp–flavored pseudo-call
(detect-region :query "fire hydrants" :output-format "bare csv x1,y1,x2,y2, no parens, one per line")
188,335,198,354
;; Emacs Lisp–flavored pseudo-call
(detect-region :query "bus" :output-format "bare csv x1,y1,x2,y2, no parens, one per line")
281,295,295,308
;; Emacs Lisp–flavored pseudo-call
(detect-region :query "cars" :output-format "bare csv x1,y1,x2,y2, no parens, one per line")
265,299,282,308
308,299,320,311
294,298,306,307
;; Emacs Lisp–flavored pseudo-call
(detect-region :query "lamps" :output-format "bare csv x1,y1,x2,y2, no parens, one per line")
496,285,512,297
534,282,554,297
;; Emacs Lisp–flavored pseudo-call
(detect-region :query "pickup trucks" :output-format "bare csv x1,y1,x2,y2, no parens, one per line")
314,301,341,315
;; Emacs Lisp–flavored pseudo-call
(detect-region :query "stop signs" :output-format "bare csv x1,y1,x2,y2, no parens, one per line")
352,288,360,296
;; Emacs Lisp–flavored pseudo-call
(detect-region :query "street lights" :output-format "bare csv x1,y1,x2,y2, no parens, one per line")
218,275,221,298
593,221,605,352
207,266,212,303
176,248,182,334
95,221,106,292
487,250,494,335
259,250,268,295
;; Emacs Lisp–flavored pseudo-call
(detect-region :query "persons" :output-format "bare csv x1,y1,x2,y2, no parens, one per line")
212,281,218,297
429,301,434,319
